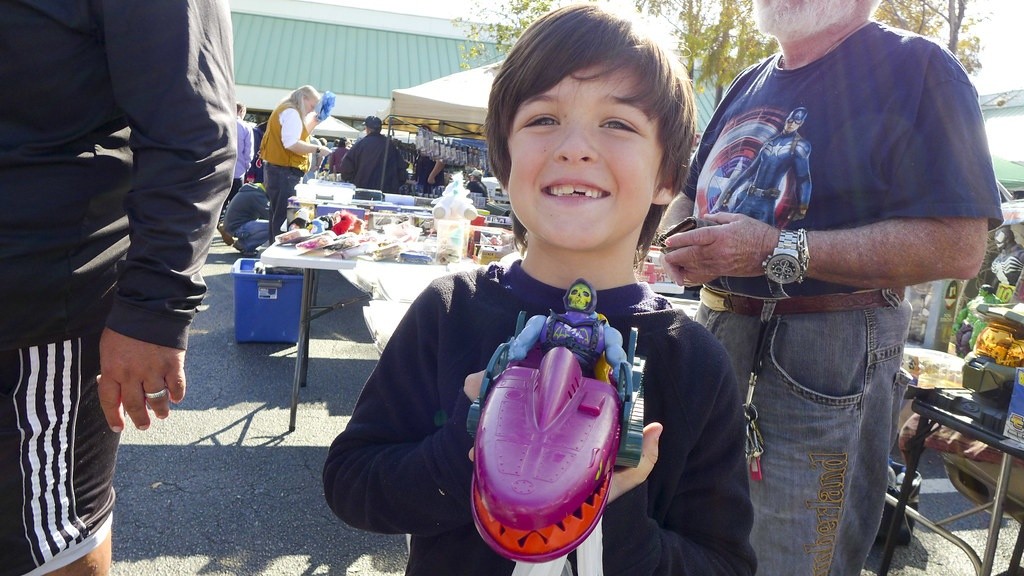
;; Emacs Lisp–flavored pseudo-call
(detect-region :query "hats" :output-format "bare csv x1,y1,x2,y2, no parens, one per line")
361,116,382,128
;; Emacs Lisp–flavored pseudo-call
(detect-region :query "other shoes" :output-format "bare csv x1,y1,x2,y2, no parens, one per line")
217,225,234,246
232,242,259,257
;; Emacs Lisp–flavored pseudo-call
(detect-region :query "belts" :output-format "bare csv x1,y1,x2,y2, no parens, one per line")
699,284,906,317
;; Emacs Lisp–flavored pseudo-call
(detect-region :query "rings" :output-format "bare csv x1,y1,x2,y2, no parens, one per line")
144,389,166,398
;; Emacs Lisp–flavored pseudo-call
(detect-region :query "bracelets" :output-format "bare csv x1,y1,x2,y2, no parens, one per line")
797,229,810,283
317,146,320,154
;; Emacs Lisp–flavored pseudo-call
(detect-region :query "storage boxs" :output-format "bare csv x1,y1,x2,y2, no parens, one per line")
229,258,304,345
294,183,356,204
637,244,674,284
1002,366,1024,445
471,244,506,265
316,205,366,221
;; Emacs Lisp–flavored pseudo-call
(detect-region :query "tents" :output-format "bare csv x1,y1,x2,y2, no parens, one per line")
381,58,506,194
305,111,360,140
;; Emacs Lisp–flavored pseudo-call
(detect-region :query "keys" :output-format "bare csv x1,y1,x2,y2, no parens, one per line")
745,413,763,471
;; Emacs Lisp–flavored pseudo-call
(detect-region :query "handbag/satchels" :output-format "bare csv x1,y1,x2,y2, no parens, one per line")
872,460,923,548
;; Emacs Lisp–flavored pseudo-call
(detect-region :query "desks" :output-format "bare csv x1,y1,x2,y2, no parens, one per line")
877,399,1024,576
260,241,484,432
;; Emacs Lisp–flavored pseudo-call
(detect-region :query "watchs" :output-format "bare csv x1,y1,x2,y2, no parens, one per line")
762,229,803,284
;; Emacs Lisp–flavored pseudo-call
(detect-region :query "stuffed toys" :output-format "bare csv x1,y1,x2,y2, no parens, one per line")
288,171,477,241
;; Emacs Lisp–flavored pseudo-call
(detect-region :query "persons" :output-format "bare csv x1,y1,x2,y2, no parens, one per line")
217,104,268,246
952,285,1003,356
658,0,1005,576
467,169,487,198
260,86,333,246
302,135,355,183
341,116,409,194
321,5,758,576
0,0,237,576
416,151,444,186
223,182,271,258
990,227,1024,294
